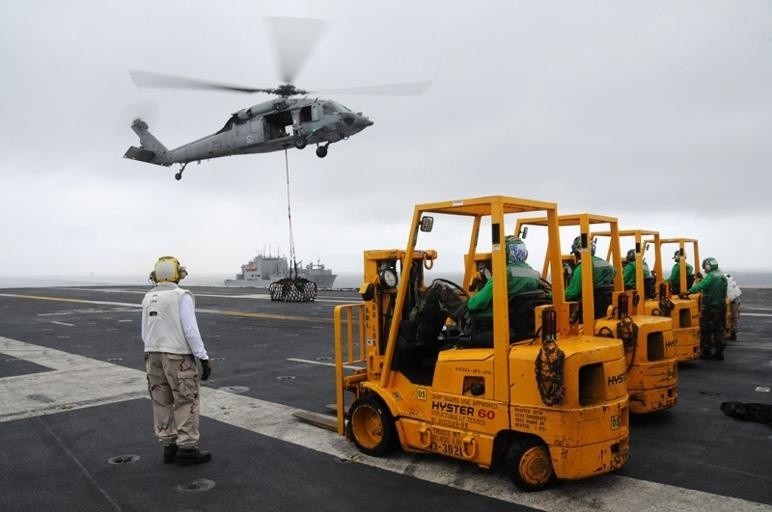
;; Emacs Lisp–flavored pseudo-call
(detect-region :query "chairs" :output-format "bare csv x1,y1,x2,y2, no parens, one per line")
459,290,546,348
568,284,614,323
625,278,656,301
671,275,694,295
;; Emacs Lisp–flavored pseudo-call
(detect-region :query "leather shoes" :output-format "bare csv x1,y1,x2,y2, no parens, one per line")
713,352,724,360
175,448,211,463
163,447,179,464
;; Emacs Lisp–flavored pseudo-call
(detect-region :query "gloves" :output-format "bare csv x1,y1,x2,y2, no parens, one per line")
201,360,211,380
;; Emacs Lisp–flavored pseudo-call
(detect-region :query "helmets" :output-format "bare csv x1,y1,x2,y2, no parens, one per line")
703,257,718,272
672,250,686,262
627,249,635,262
155,257,180,282
570,236,596,260
506,235,528,263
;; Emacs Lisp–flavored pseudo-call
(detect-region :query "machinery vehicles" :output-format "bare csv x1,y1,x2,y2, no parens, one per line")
293,195,632,492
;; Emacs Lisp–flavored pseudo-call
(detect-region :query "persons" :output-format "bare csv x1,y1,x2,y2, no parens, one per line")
141,255,212,467
679,257,728,361
664,249,693,294
404,235,541,344
725,274,743,341
623,249,649,291
540,235,617,300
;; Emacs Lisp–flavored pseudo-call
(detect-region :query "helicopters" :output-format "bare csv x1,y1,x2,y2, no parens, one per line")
124,18,431,181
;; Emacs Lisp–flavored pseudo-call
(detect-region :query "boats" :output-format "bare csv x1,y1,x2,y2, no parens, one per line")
224,244,337,290
